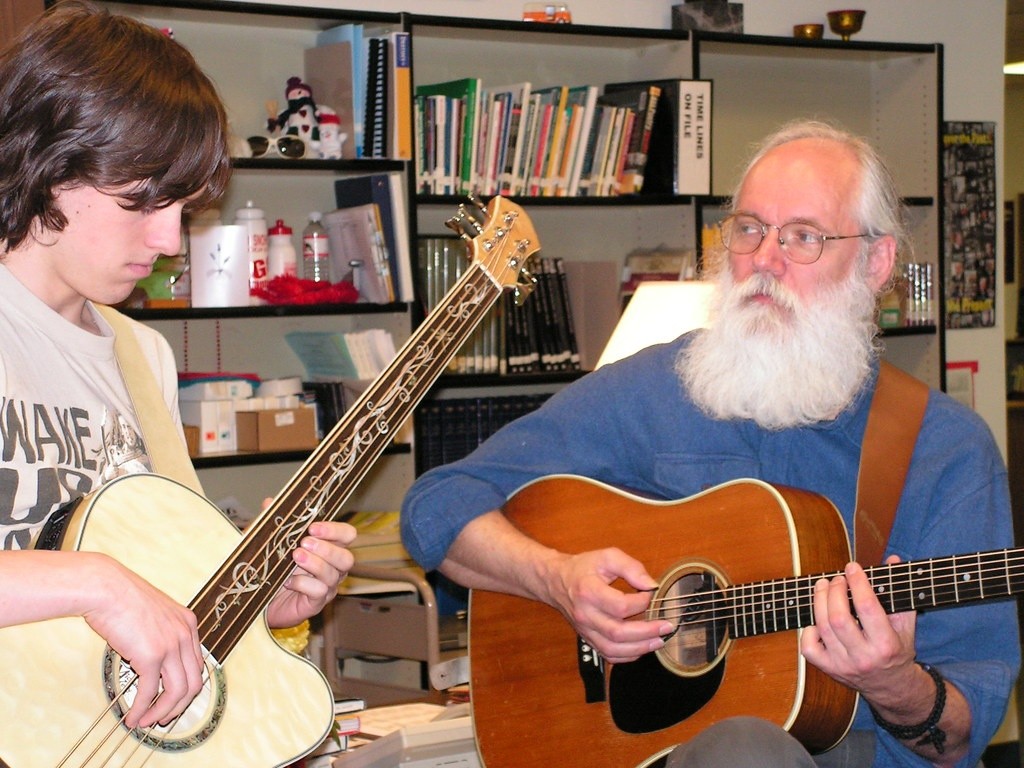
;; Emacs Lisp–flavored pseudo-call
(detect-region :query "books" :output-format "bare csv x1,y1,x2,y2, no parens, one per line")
875,261,933,328
414,78,686,477
299,679,366,759
321,203,395,302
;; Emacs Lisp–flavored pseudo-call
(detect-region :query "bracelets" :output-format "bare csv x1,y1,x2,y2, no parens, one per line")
870,661,947,755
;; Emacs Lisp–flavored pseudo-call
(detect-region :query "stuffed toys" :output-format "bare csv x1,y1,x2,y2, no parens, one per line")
266,77,348,159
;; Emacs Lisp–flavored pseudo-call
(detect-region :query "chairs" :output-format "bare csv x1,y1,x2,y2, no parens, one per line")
318,563,441,696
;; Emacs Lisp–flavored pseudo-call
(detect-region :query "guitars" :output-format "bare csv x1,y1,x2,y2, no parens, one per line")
0,193,543,767
467,472,1024,768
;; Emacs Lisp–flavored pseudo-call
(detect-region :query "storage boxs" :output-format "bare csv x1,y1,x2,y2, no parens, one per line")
179,399,321,456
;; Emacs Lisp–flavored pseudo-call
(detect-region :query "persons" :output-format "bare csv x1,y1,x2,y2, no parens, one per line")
398,124,1019,768
0,0,358,767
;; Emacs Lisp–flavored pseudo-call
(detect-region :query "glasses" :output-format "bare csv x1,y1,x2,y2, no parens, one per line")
718,213,881,264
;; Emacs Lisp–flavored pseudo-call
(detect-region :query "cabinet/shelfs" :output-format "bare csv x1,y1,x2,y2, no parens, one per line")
42,0,947,611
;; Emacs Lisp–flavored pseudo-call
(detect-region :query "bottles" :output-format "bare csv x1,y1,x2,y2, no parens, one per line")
235,199,267,306
188,208,223,228
301,211,330,283
263,219,297,283
